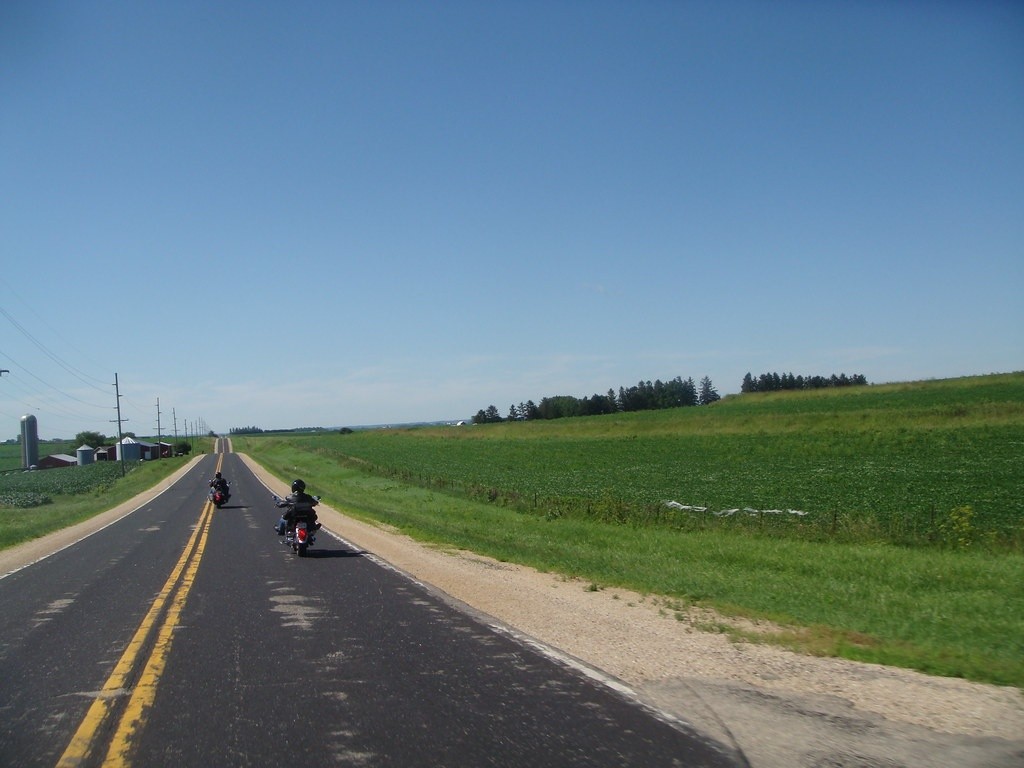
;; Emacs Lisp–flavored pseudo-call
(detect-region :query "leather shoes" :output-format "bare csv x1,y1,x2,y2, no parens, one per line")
273,524,285,536
313,523,321,530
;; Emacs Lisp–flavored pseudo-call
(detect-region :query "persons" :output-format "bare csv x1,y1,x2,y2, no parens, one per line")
273,479,321,532
208,472,231,500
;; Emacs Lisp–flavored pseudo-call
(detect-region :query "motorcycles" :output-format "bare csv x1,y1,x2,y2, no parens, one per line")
208,480,232,509
272,493,324,556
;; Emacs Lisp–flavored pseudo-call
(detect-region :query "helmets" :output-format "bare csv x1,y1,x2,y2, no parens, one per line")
216,472,221,478
292,479,305,493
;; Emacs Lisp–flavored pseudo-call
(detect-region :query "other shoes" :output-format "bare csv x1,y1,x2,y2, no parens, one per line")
227,494,231,499
